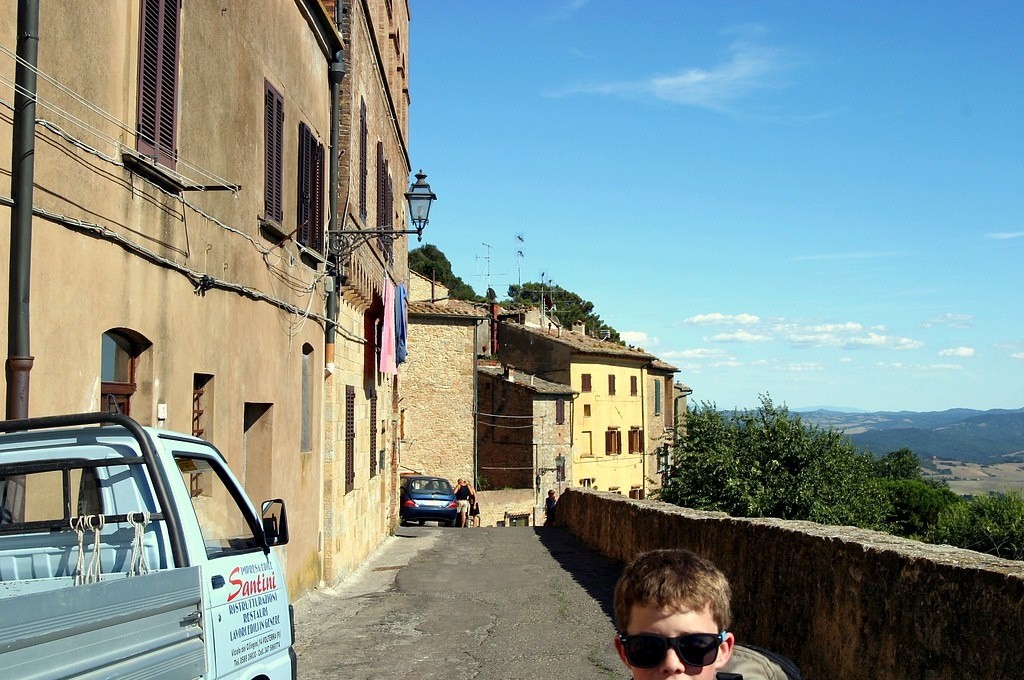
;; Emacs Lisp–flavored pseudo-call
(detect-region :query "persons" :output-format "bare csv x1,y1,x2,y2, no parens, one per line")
544,490,558,517
464,481,480,528
453,479,472,528
610,547,735,679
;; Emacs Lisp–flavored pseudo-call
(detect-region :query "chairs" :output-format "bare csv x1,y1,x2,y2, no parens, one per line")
425,483,434,491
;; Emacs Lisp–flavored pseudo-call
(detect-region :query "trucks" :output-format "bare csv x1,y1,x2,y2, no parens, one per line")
0,392,298,680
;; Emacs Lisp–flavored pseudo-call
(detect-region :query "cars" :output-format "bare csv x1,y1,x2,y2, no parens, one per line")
400,474,460,527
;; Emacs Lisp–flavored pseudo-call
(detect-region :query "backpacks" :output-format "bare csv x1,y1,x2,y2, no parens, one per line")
712,643,806,680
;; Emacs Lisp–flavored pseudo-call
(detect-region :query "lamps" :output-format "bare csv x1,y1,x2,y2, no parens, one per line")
325,169,437,274
536,453,565,476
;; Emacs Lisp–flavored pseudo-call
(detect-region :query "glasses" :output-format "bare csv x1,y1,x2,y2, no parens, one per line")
618,630,726,668
